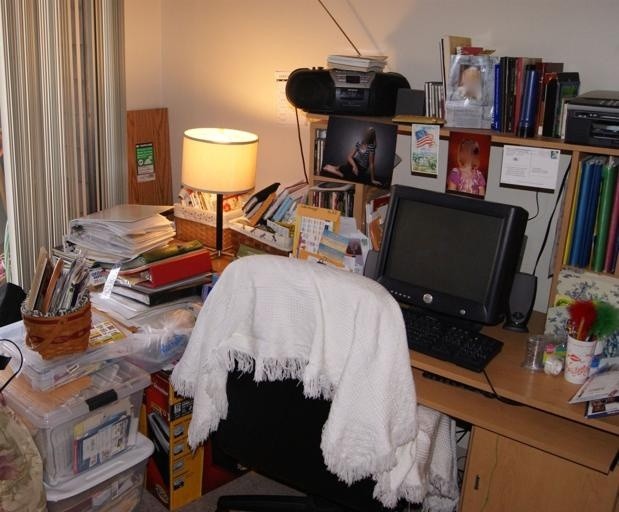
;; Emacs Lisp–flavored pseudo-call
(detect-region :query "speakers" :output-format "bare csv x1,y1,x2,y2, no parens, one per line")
502,272,537,333
363,250,379,279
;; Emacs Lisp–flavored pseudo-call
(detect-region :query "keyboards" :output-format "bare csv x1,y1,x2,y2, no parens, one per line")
400,306,504,374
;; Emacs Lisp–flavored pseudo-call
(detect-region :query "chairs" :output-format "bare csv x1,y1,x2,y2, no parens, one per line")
168,253,459,511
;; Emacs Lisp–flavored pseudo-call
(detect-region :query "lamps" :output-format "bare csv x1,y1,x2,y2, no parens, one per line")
180,127,259,261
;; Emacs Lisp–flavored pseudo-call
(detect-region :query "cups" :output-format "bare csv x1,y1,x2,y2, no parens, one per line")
564,333,598,385
522,335,545,370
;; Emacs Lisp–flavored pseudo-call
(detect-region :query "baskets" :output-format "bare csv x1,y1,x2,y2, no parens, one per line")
174,204,244,253
21,301,92,361
229,216,293,258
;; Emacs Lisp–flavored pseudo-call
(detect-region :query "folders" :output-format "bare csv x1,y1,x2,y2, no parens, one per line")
118,248,212,288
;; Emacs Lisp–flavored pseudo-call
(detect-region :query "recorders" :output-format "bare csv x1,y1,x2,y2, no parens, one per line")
285,67,412,117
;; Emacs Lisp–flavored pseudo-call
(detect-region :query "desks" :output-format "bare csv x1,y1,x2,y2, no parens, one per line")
205,111,619,512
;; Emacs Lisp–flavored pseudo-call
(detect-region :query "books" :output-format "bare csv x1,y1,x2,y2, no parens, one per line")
562,154,616,280
50,203,213,307
242,181,309,226
327,55,389,82
424,35,580,138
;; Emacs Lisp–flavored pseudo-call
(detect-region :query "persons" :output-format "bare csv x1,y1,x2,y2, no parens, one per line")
324,126,384,186
447,135,486,196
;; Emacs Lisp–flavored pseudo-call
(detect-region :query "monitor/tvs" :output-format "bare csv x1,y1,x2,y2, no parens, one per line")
374,184,529,329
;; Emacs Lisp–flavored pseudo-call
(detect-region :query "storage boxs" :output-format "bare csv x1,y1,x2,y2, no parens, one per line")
142,368,251,512
40,432,153,512
0,358,151,487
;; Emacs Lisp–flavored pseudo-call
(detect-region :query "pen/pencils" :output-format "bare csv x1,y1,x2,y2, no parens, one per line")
567,301,619,342
